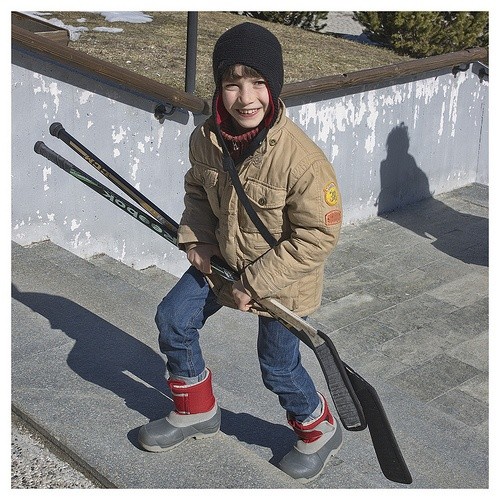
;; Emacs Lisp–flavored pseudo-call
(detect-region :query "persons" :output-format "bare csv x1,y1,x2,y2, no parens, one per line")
136,21,346,486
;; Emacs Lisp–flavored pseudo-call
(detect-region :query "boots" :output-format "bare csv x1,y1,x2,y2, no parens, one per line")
279,391,344,483
138,367,221,453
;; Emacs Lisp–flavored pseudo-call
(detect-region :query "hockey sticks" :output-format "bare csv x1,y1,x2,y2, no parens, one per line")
33,141,368,433
50,123,414,485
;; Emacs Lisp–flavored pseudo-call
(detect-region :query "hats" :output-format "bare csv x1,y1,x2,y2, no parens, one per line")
212,21,282,129
